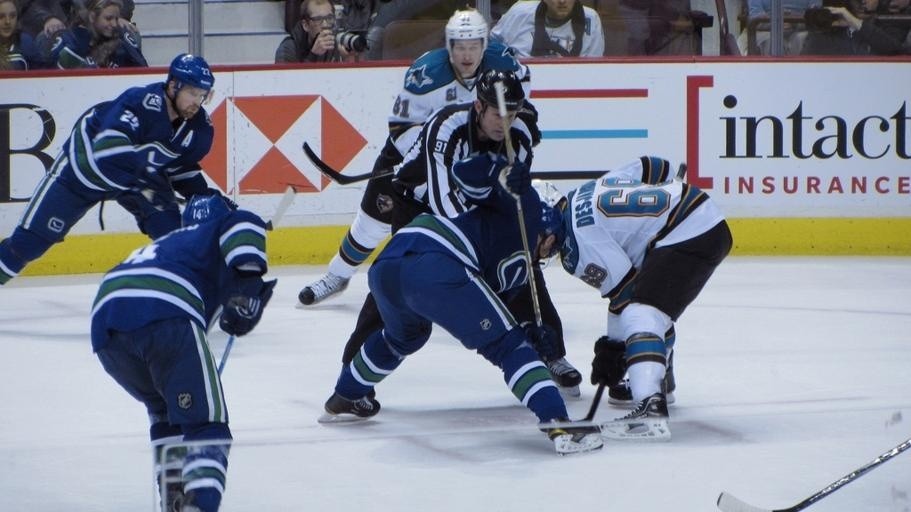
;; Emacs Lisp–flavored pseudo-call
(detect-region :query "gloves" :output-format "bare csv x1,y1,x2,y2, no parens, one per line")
525,318,563,363
219,277,277,338
498,159,530,200
141,180,183,212
591,336,625,388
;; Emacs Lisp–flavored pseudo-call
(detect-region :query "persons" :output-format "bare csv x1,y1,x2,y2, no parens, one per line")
533,154,735,427
339,68,585,388
323,154,603,442
272,0,910,65
298,8,532,304
89,187,268,511
0,1,149,70
0,53,222,286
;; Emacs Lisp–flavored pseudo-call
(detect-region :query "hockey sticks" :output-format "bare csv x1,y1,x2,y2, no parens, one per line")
127,176,298,230
562,384,606,428
717,439,909,512
302,141,403,186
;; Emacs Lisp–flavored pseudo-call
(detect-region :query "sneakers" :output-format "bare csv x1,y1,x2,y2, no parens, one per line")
608,367,675,400
539,417,601,440
299,272,349,305
546,357,582,387
616,393,669,420
326,392,381,418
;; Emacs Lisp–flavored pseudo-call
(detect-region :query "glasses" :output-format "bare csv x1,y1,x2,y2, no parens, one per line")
308,13,336,26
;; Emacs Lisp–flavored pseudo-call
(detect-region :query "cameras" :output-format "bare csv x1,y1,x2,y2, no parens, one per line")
324,28,366,53
803,6,833,30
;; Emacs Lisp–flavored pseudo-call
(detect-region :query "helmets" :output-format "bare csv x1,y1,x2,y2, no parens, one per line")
181,192,230,227
445,9,489,64
166,52,215,92
475,68,525,111
539,202,566,257
531,178,564,208
